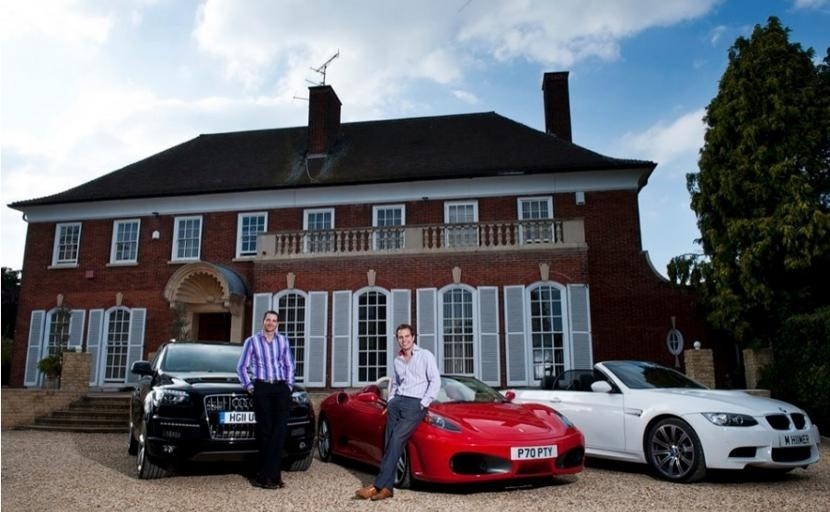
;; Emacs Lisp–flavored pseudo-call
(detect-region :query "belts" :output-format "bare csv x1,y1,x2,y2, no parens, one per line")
395,394,418,399
256,381,283,385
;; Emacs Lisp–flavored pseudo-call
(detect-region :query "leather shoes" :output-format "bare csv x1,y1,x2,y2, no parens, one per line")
252,481,285,489
355,485,393,500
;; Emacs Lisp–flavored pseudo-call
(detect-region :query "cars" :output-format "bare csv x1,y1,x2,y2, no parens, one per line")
126,337,317,480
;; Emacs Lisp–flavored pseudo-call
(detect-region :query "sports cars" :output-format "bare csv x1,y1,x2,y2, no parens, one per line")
497,358,822,484
317,375,586,489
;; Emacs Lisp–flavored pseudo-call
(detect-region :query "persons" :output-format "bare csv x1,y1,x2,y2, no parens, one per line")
235,310,296,488
354,323,443,501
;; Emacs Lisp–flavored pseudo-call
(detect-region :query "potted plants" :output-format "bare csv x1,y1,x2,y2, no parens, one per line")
37,357,60,389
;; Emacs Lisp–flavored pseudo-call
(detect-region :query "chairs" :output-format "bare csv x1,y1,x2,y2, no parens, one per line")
579,374,594,391
